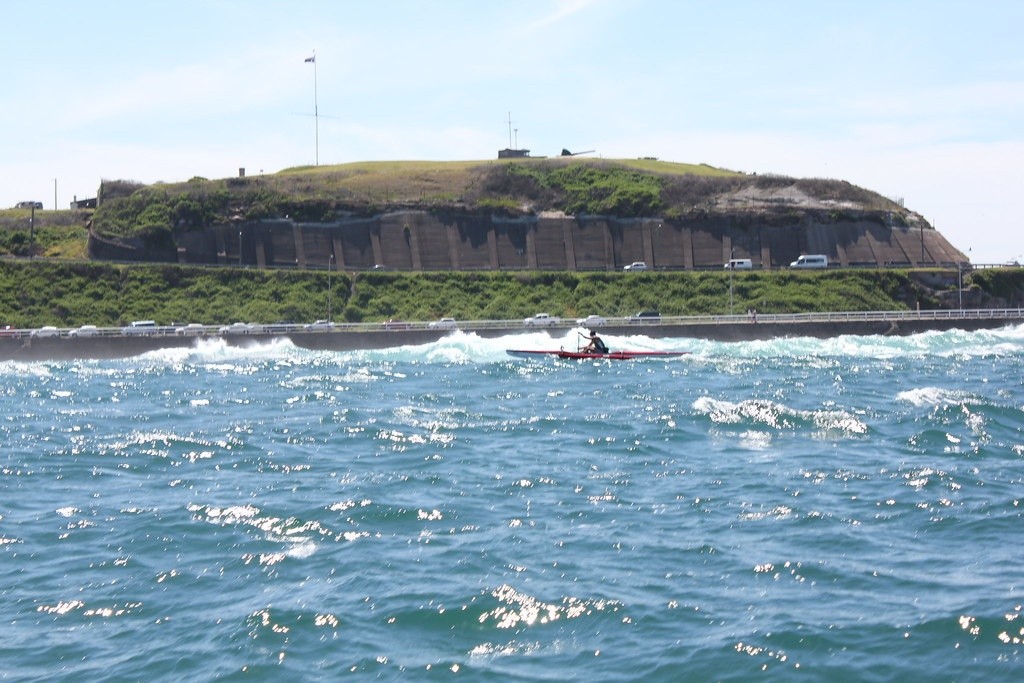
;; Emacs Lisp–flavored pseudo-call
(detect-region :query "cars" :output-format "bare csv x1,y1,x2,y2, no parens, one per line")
381,318,413,330
67,325,99,338
304,319,334,331
577,315,606,327
428,317,456,330
30,326,61,339
371,264,387,271
176,323,206,336
219,322,254,336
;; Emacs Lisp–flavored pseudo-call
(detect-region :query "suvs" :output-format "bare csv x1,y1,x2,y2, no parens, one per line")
623,261,646,271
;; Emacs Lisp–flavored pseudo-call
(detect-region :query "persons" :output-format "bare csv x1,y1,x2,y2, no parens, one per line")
582,331,605,353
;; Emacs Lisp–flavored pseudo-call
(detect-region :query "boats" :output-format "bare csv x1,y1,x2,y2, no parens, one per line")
506,347,695,361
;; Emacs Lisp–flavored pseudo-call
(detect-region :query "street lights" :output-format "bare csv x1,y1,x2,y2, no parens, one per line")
328,255,334,322
957,247,972,309
305,50,319,167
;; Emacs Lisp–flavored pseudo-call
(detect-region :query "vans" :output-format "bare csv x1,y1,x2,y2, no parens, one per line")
725,258,752,271
14,201,44,209
624,311,662,323
789,254,828,269
120,320,157,336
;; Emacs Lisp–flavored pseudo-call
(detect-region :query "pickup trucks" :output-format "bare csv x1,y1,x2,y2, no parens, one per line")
523,313,561,327
